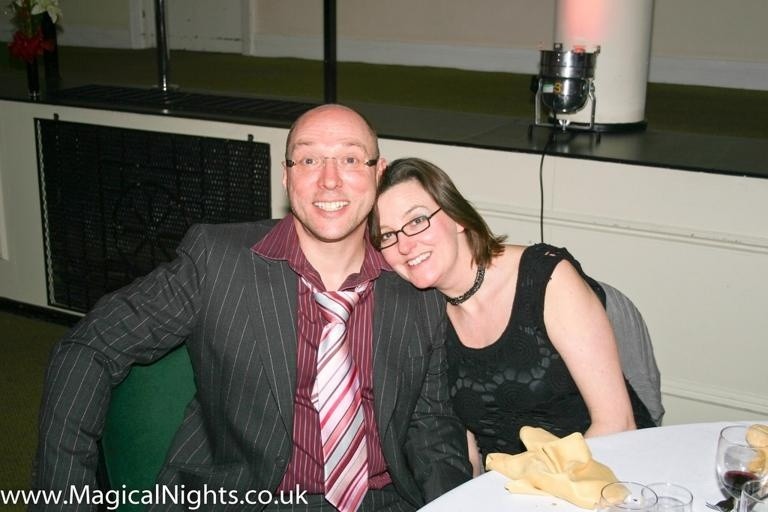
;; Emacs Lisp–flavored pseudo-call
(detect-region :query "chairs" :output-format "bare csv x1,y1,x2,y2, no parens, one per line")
53,322,199,511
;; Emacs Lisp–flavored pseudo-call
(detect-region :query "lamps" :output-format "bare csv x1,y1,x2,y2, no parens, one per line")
528,41,604,136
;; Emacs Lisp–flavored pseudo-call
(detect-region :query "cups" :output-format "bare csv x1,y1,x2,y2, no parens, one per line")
738,479,767,509
597,480,694,512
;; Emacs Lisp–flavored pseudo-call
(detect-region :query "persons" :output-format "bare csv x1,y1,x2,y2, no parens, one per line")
32,103,472,511
368,156,657,481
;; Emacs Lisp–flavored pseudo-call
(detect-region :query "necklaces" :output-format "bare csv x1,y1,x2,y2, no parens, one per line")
444,259,486,306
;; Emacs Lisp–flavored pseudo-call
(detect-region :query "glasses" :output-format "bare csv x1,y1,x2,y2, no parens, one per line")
371,207,443,252
286,156,377,169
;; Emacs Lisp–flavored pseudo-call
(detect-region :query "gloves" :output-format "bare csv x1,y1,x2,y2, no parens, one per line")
744,423,768,478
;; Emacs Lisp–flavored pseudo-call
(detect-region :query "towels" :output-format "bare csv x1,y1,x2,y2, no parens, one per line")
745,422,768,451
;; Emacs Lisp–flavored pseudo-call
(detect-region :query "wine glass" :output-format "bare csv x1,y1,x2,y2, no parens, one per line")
714,422,768,512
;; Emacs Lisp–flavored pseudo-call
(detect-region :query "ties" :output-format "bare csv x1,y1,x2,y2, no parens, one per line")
300,277,375,508
